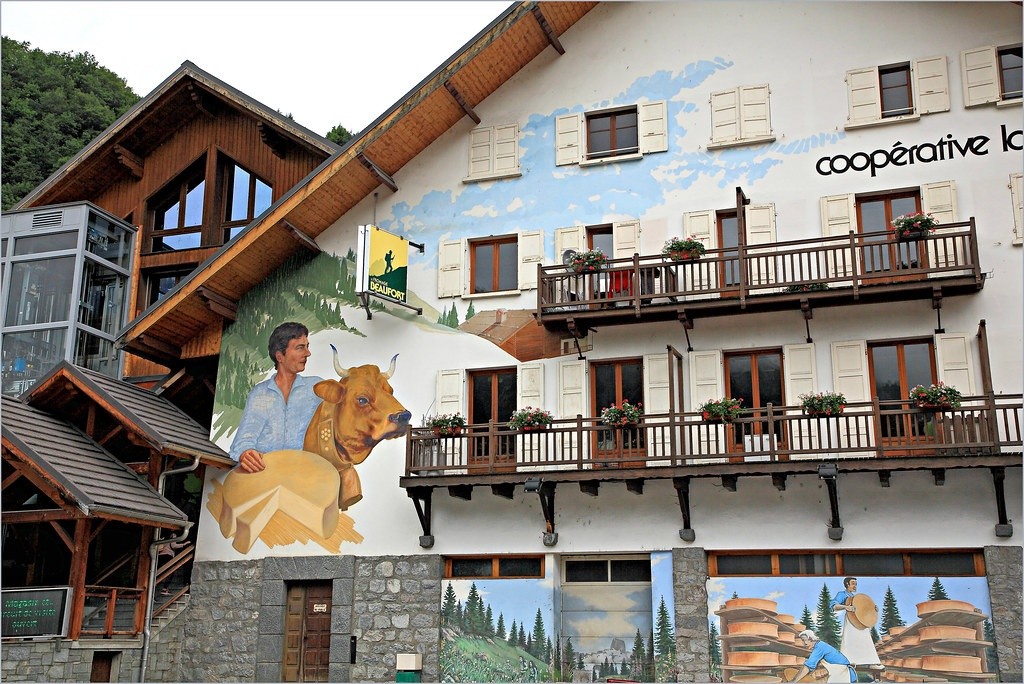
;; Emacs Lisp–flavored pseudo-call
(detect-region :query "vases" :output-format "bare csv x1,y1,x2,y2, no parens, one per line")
916,396,952,407
807,405,844,413
433,426,461,435
578,264,595,271
519,423,547,430
671,251,690,260
703,411,731,419
895,229,927,240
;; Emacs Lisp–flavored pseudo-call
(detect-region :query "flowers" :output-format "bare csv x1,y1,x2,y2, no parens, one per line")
697,398,743,425
909,382,961,408
432,411,467,435
508,406,555,430
891,212,939,235
798,391,846,416
661,235,708,262
566,247,606,272
601,400,644,428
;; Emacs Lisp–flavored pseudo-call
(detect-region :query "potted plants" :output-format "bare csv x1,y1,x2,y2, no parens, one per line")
785,283,828,292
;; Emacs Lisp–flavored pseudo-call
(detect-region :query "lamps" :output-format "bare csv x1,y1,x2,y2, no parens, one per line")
522,476,545,493
816,463,840,481
